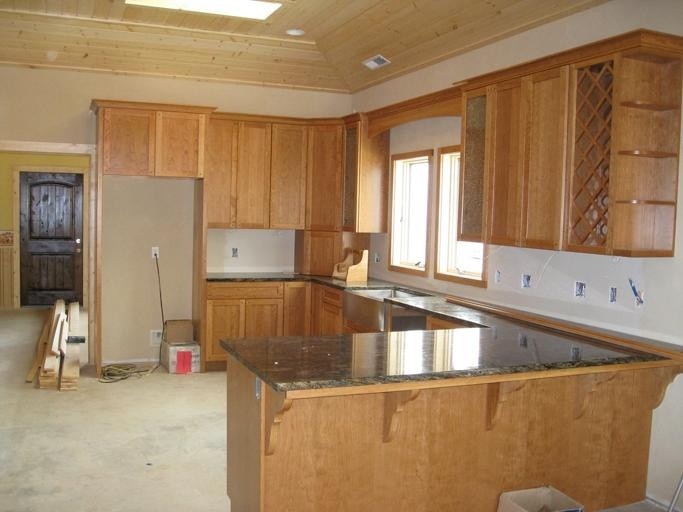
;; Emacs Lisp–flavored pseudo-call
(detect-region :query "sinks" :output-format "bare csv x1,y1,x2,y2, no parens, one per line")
343,289,427,331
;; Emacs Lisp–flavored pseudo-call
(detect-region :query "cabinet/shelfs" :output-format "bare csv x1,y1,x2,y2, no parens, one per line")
89,97,387,274
192,278,470,375
225,352,682,511
455,32,681,257
